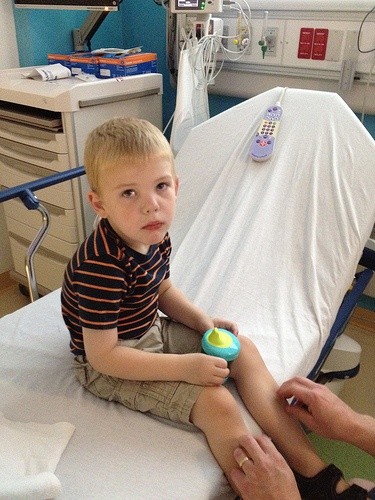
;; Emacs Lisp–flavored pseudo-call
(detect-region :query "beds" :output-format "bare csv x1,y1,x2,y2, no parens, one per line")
0,89,375,500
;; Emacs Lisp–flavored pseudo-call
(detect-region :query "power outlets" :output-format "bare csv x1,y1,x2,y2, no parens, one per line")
186,17,228,53
297,28,359,62
261,26,278,57
235,26,253,55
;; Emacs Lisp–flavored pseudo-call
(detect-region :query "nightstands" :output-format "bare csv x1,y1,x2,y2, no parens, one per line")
0,66,163,299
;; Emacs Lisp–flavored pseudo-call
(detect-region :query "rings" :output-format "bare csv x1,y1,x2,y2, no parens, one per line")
239,456,249,468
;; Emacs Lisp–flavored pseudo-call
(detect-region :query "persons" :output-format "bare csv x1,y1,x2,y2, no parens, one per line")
230,375,375,500
61,114,375,499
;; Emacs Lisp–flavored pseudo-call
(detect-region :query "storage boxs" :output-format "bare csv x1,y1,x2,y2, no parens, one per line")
48,50,157,80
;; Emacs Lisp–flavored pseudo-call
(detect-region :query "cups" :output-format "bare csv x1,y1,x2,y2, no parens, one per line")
201,327,240,384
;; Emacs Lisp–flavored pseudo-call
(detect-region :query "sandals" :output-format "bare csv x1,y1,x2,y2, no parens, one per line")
291,463,375,500
